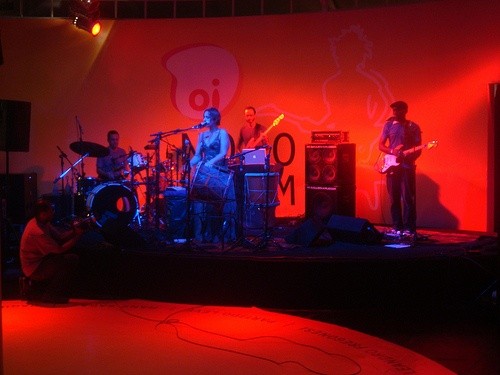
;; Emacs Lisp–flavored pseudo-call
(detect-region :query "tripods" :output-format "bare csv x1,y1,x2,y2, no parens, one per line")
145,126,286,251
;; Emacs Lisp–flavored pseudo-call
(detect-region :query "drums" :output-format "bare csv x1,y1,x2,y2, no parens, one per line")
75,176,138,229
189,160,237,201
245,172,282,229
124,152,148,175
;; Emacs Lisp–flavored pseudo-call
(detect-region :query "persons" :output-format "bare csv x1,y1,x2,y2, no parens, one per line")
20,197,82,299
183,108,237,243
379,101,422,225
97,130,129,182
237,106,268,155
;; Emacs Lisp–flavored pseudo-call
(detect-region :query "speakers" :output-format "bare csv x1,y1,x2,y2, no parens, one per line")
0,172,38,224
304,142,356,221
0,99,32,153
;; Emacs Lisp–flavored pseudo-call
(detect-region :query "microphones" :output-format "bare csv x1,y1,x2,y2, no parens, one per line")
195,122,207,128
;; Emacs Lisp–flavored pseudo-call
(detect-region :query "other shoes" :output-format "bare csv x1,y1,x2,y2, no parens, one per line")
387,230,400,237
397,230,414,238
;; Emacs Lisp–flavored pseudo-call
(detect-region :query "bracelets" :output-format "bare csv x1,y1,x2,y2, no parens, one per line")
391,150,392,153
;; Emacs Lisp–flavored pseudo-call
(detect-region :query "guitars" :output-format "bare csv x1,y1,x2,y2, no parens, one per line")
237,113,285,154
377,139,439,175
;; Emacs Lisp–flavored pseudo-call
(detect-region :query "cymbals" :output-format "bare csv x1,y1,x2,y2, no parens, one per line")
69,141,112,157
